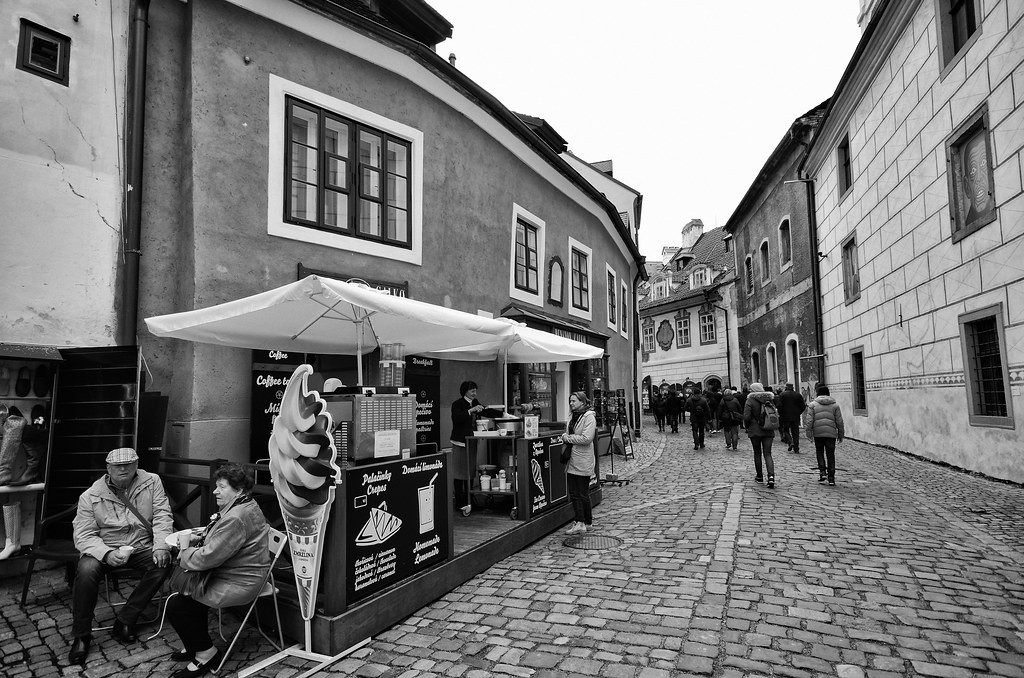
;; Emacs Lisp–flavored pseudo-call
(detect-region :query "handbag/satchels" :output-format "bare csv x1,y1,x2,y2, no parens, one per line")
560,443,572,464
168,563,213,596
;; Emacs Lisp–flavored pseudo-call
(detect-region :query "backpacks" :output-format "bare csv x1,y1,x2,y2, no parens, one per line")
752,401,780,430
730,410,743,422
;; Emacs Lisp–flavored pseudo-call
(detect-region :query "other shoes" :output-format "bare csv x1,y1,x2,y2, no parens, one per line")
173,648,223,678
658,427,740,451
755,477,763,483
565,522,586,534
795,450,799,453
0,364,51,427
818,475,828,484
171,650,195,661
829,479,836,486
767,476,774,487
586,525,594,531
788,443,794,451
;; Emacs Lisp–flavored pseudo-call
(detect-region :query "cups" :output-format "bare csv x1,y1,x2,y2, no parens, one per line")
178,529,193,549
119,546,134,563
476,420,489,431
497,429,508,436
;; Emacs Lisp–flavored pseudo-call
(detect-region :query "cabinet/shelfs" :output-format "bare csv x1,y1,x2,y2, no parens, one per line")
511,369,551,400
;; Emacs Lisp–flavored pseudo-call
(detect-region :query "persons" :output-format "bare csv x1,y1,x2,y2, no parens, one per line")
562,392,596,535
1,415,27,560
652,383,806,453
743,382,775,489
805,383,844,486
166,465,270,678
68,448,173,665
450,381,513,510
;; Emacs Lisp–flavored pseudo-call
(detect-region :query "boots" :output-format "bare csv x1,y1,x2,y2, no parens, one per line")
0,415,27,486
8,425,47,486
0,502,22,560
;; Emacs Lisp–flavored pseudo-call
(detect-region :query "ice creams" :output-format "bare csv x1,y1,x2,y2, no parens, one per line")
269,365,338,622
531,459,544,494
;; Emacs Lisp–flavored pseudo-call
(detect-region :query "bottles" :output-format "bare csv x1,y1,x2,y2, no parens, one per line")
480,475,491,490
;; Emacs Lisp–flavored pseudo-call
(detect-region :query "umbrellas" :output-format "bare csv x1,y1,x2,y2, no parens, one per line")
144,273,526,387
498,317,605,413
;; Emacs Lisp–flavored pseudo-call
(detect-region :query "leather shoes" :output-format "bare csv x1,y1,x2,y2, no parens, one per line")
68,634,91,664
112,619,137,642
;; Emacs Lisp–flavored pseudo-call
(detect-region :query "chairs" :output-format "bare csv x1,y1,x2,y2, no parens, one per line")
71,568,164,632
18,500,120,610
209,527,288,675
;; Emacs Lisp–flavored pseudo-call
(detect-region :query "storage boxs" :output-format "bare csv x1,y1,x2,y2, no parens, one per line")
473,430,501,437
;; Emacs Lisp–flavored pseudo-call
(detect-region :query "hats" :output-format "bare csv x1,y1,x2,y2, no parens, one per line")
106,448,140,466
750,383,764,392
784,382,794,389
817,386,830,396
